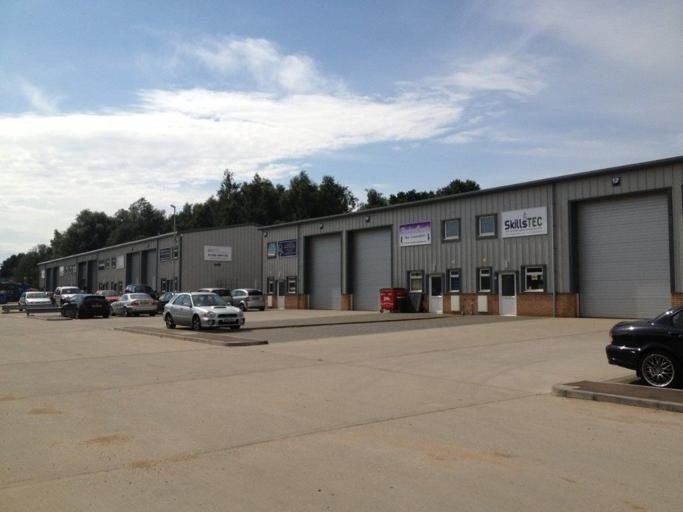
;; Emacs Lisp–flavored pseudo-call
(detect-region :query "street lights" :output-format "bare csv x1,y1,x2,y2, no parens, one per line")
169,205,175,290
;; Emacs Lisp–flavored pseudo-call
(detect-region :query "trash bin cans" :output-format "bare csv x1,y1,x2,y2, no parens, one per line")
380,288,409,313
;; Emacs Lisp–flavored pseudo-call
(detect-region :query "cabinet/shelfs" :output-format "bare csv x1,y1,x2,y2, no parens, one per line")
378,287,405,313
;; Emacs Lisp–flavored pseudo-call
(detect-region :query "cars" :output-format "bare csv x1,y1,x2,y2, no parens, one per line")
161,290,247,331
604,304,682,389
0,281,265,318
